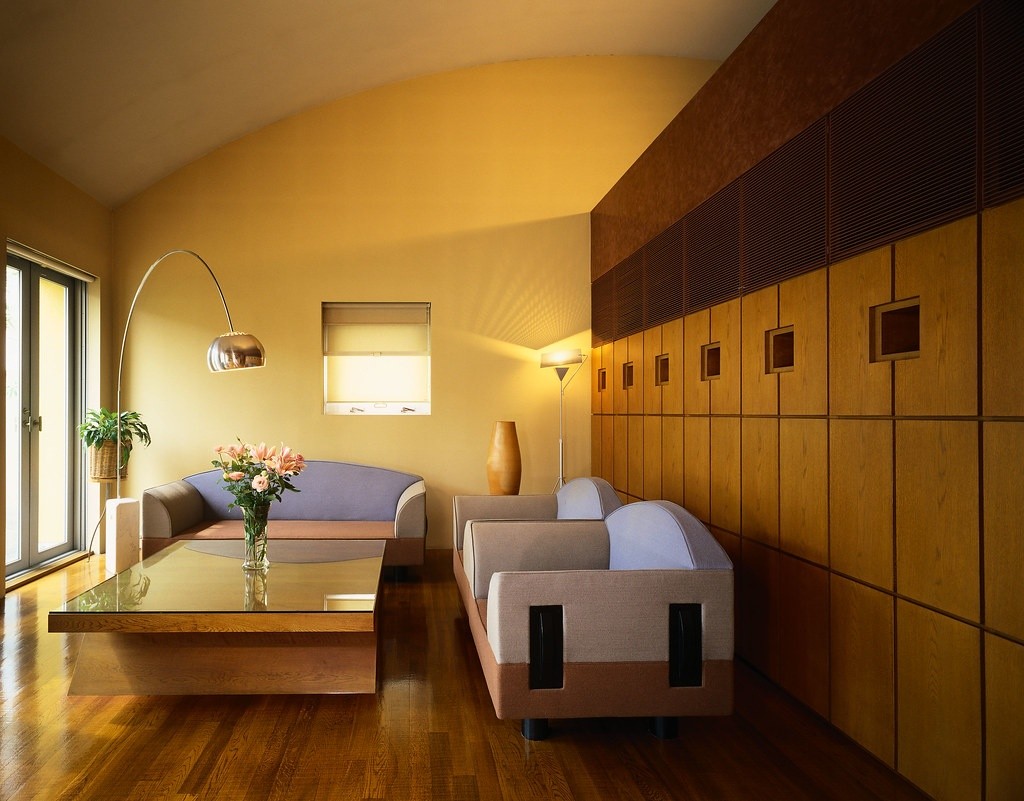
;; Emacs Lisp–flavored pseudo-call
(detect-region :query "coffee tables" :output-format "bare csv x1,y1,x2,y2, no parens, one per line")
47,540,388,696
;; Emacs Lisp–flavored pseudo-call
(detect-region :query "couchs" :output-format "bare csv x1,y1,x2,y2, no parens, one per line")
451,477,737,742
142,460,427,583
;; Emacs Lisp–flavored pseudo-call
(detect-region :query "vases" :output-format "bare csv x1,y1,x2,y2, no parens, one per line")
237,503,271,569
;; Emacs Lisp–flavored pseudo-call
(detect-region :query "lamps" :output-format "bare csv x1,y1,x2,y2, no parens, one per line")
105,250,269,573
540,349,587,494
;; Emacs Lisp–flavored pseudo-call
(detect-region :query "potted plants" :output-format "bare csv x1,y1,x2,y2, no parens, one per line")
73,409,151,478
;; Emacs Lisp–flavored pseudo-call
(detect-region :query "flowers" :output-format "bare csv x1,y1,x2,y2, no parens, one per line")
211,436,308,567
